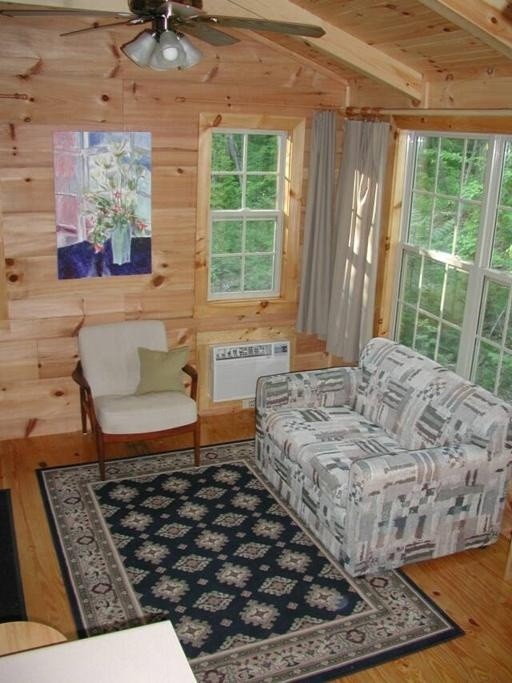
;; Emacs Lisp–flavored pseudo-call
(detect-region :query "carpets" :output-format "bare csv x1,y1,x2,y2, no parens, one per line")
0,488,31,624
34,437,466,683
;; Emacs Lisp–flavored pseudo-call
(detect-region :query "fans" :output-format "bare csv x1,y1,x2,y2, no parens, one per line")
0,1,326,46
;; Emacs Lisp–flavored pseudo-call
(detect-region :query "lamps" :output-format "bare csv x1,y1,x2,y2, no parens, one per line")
120,16,203,72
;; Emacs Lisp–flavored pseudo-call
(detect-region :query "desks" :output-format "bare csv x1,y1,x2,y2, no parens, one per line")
0,618,199,683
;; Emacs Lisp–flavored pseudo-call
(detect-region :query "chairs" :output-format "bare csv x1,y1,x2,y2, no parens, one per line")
72,321,201,481
0,620,69,660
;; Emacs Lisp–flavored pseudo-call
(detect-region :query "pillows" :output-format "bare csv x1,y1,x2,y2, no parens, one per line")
136,347,190,394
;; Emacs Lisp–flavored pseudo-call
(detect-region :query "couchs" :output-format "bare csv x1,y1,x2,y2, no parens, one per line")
255,336,512,577
